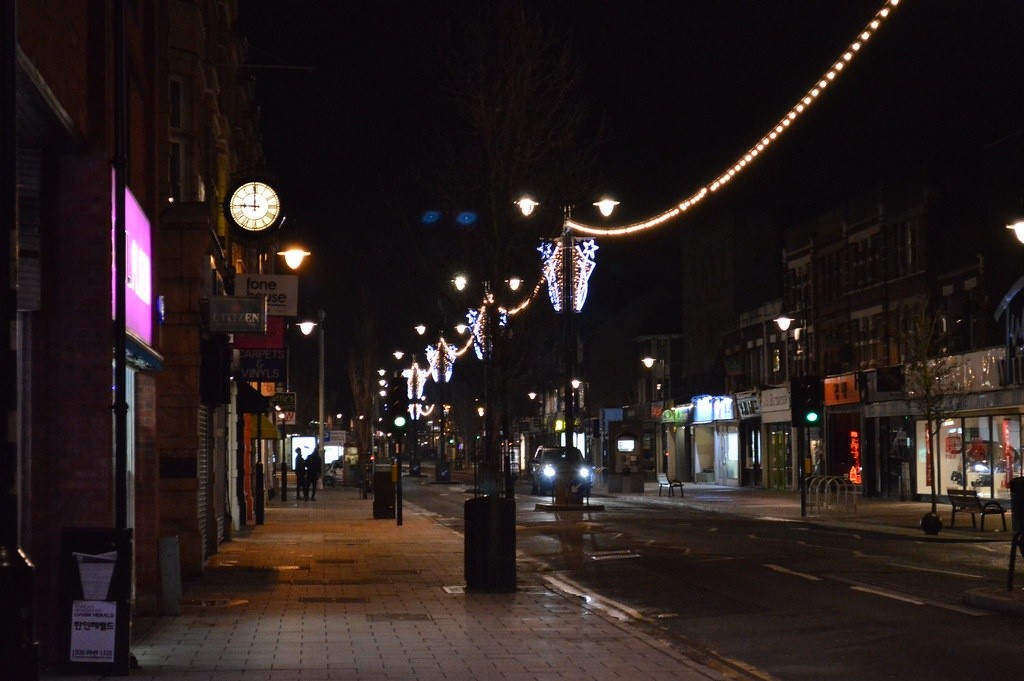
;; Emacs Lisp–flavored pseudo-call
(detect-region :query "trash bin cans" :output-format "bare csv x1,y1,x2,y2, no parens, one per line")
62,528,132,675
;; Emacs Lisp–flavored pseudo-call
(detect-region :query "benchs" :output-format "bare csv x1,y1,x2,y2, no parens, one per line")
947,489,1007,533
656,472,684,497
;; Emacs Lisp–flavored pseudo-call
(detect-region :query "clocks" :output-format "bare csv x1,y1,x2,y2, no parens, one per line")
222,177,287,249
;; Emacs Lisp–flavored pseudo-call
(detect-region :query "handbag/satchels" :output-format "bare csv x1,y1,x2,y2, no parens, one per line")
316,478,323,490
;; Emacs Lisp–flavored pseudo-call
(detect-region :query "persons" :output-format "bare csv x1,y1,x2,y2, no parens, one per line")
295,447,321,501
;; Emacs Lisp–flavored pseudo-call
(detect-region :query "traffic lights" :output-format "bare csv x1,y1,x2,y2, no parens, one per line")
803,375,822,427
387,376,409,431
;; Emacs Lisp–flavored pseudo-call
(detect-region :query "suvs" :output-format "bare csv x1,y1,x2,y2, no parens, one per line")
525,444,595,497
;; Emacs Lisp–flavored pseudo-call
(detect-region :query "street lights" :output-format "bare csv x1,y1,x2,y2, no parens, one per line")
376,350,428,478
511,192,621,512
450,273,525,498
415,324,469,485
774,312,808,519
639,352,669,480
